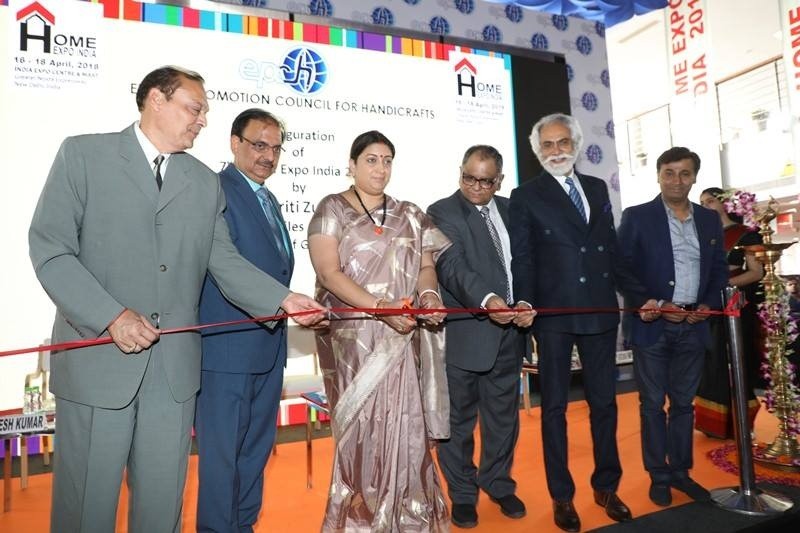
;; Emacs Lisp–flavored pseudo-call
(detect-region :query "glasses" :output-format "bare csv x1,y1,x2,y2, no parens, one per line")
462,171,496,189
240,134,285,155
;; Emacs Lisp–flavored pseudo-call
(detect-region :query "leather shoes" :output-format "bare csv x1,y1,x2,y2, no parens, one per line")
552,500,582,533
593,489,633,524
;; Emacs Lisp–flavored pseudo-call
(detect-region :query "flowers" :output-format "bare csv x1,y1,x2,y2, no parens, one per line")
724,189,800,470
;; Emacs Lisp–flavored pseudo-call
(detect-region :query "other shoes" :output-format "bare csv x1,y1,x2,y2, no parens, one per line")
672,478,710,503
450,503,479,528
649,481,672,506
489,494,525,519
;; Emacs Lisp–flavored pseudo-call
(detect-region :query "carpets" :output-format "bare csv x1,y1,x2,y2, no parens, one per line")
0,391,800,533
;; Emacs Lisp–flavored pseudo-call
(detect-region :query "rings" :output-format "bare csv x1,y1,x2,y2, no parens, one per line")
130,343,137,352
440,316,444,323
398,327,406,333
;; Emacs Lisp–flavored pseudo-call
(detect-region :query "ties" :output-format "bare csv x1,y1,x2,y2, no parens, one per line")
257,188,289,266
479,207,511,305
565,176,586,222
153,155,166,192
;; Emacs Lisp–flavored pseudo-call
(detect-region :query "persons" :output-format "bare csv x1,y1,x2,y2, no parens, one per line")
700,187,800,440
307,128,453,533
425,145,534,528
196,108,294,533
512,113,661,533
29,65,330,533
615,147,728,507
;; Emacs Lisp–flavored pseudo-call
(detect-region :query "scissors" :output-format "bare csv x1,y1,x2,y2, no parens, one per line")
402,297,432,322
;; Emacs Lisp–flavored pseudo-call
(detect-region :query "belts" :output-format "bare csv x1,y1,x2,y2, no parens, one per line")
678,304,693,312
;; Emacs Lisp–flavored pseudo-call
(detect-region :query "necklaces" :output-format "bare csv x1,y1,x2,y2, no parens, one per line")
352,184,386,234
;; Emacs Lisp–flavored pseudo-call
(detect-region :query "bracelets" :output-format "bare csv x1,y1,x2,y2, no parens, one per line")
420,289,441,303
375,298,387,320
